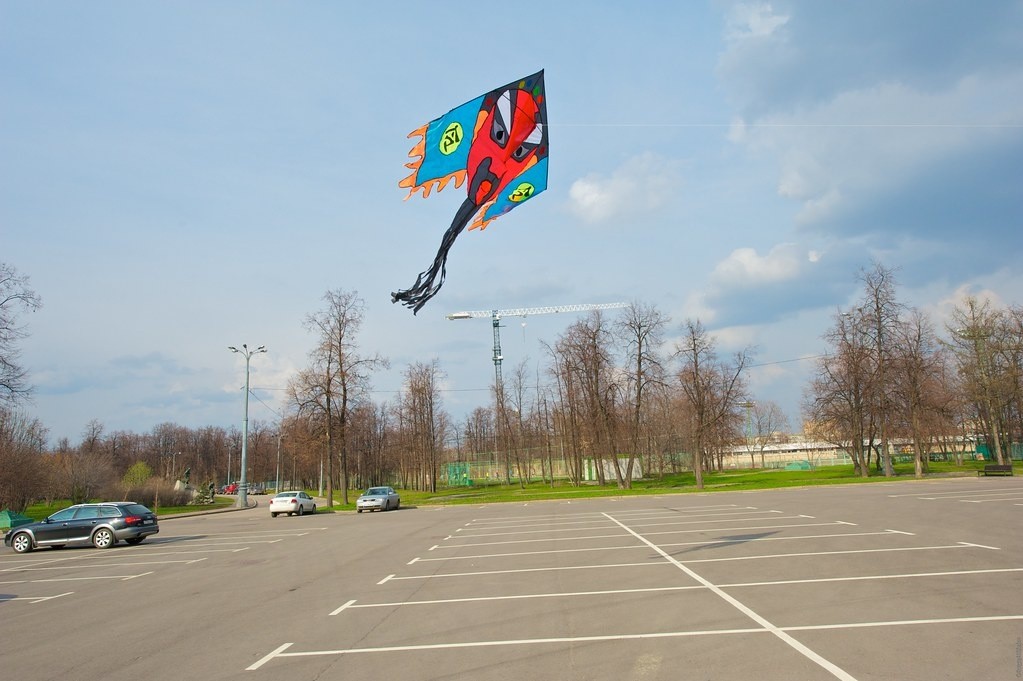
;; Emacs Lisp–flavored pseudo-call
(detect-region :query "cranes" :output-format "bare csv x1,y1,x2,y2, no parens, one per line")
445,301,633,436
731,399,755,444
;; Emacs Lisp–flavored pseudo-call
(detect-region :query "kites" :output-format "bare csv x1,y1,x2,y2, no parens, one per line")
391,68,549,315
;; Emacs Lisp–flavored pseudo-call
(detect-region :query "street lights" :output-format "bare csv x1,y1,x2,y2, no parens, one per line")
173,451,182,476
228,344,267,507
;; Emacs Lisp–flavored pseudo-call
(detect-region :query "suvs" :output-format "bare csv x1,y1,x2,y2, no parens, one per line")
5,501,159,554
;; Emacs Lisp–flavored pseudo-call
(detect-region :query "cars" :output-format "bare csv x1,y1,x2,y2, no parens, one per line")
356,486,400,513
270,491,316,517
218,481,262,496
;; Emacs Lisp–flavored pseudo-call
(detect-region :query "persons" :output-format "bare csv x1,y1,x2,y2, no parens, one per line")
184,468,191,484
209,482,215,500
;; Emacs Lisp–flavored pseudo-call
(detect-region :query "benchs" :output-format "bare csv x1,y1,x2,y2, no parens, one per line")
975,465,1013,477
